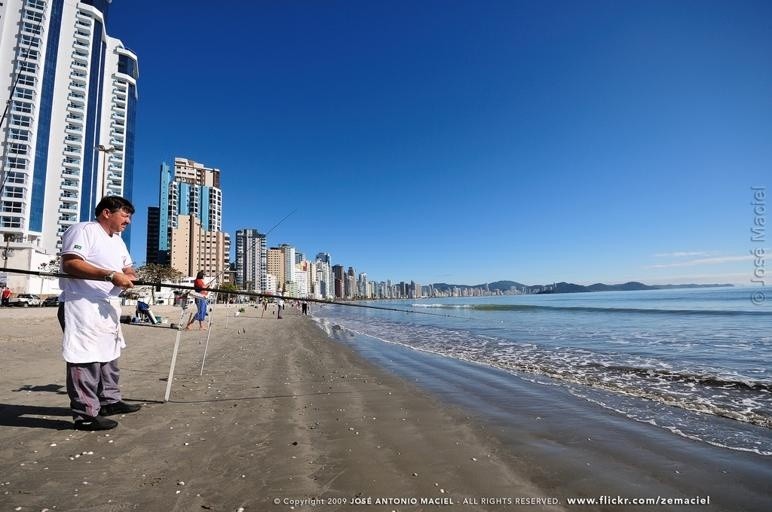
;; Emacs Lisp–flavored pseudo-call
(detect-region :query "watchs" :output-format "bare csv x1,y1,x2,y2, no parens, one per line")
103,270,116,282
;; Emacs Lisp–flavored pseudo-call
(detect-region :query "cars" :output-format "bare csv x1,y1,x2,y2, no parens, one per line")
119,289,149,300
43,298,58,306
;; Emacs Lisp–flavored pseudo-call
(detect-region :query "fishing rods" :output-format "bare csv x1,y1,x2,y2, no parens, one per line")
0,268,485,321
209,208,296,285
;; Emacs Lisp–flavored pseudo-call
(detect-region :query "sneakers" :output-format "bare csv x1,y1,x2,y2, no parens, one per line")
99,401,142,417
75,414,117,431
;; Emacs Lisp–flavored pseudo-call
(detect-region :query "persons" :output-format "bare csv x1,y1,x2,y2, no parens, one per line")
258,295,310,317
59,196,141,430
186,271,210,331
174,292,186,309
0,286,12,307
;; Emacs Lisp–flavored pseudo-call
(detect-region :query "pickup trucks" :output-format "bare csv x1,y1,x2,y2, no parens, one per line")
9,292,43,308
